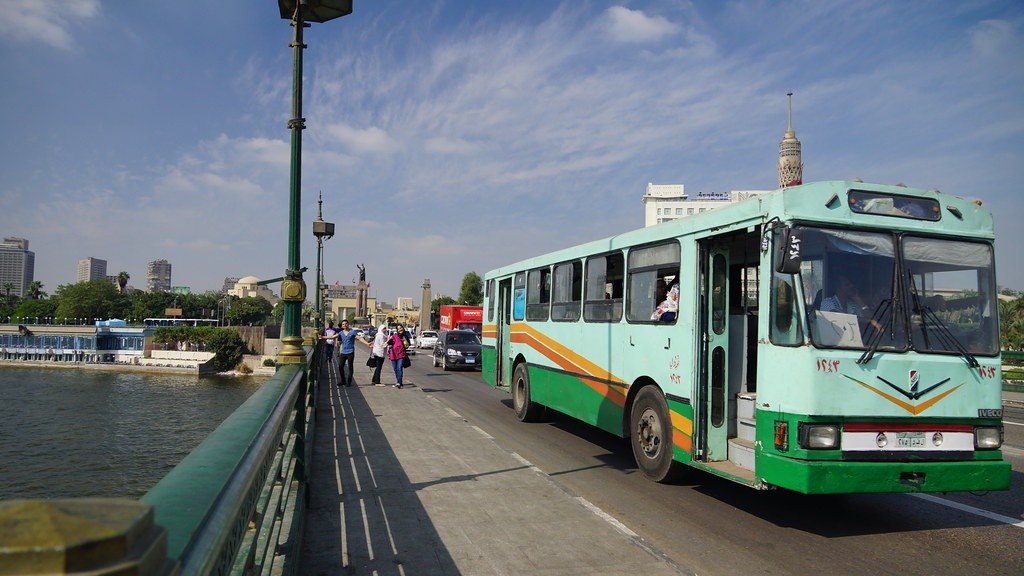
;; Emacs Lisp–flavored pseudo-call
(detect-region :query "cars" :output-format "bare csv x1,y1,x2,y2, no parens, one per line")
416,330,440,349
351,328,416,357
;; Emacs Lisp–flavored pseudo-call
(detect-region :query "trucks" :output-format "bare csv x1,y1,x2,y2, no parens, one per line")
440,305,483,339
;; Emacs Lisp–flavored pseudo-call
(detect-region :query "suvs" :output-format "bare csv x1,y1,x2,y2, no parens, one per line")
433,330,482,371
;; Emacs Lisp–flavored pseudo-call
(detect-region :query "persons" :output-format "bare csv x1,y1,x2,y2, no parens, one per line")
372,324,411,389
318,320,373,387
650,280,679,321
866,262,914,331
411,324,419,348
820,275,886,338
324,322,344,363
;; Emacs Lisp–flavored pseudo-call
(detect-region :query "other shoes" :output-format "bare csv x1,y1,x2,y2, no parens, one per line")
325,357,332,363
392,383,402,389
337,382,346,385
347,383,350,387
373,382,385,387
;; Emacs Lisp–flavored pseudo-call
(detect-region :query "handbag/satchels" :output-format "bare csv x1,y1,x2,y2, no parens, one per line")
366,357,377,367
403,336,409,349
402,355,411,368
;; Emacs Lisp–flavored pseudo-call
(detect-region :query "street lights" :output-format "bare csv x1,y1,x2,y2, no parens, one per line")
217,300,223,321
313,220,336,341
275,0,352,367
173,295,179,319
7,316,87,325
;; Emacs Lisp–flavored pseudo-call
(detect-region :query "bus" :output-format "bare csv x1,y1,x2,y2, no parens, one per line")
481,180,1011,495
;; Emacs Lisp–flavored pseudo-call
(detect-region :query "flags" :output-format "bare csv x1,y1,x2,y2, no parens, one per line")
335,280,339,287
367,282,370,287
